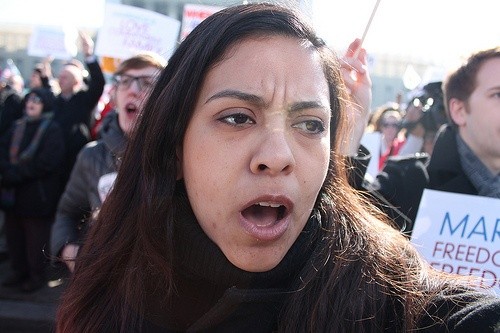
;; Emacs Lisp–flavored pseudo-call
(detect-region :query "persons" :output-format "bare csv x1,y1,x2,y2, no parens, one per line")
51,2,500,333
358,80,448,184
47,50,170,276
0,86,68,292
333,37,500,241
0,30,118,170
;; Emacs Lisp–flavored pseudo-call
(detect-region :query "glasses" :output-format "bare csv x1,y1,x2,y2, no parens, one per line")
111,71,157,92
26,95,42,104
